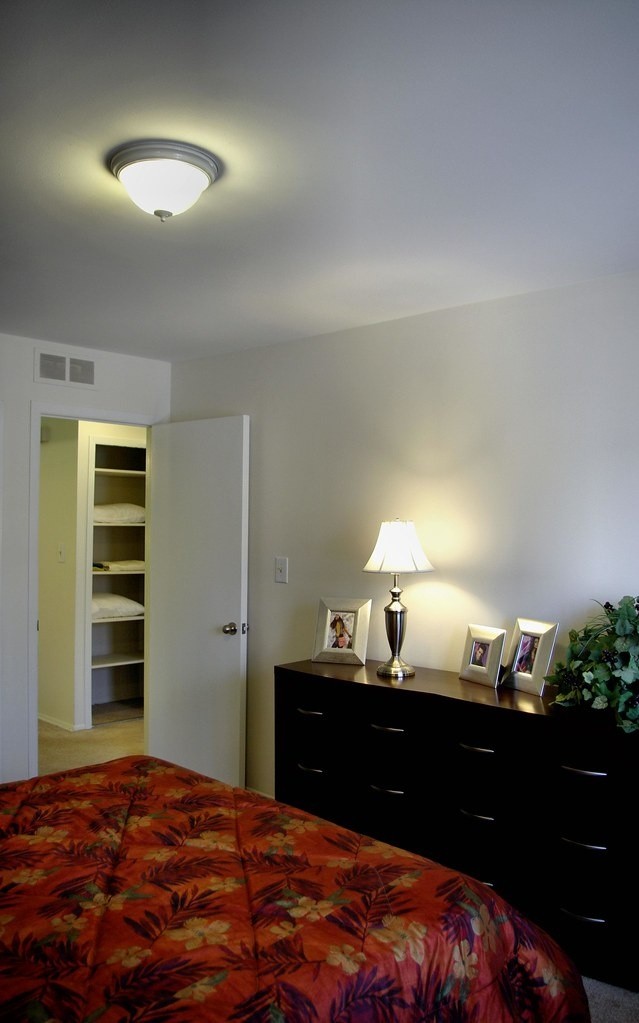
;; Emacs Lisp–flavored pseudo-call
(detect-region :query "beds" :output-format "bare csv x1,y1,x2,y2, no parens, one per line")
0,754,593,1023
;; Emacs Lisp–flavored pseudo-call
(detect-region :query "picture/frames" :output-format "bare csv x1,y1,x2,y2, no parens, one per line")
459,624,507,689
502,617,561,697
311,596,372,666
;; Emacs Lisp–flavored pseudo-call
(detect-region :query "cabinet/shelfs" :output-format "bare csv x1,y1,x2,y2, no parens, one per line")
274,659,639,994
39,415,148,733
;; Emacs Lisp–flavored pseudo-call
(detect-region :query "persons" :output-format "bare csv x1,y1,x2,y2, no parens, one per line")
517,637,539,674
330,612,352,649
471,644,487,667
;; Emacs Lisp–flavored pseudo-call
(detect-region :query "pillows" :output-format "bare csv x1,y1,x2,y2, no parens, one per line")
93,503,146,523
91,591,144,619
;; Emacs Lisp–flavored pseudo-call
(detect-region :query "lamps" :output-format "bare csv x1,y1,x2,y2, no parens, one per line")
361,517,436,678
109,138,222,223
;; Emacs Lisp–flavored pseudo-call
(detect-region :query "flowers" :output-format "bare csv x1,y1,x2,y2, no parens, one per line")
543,596,639,733
